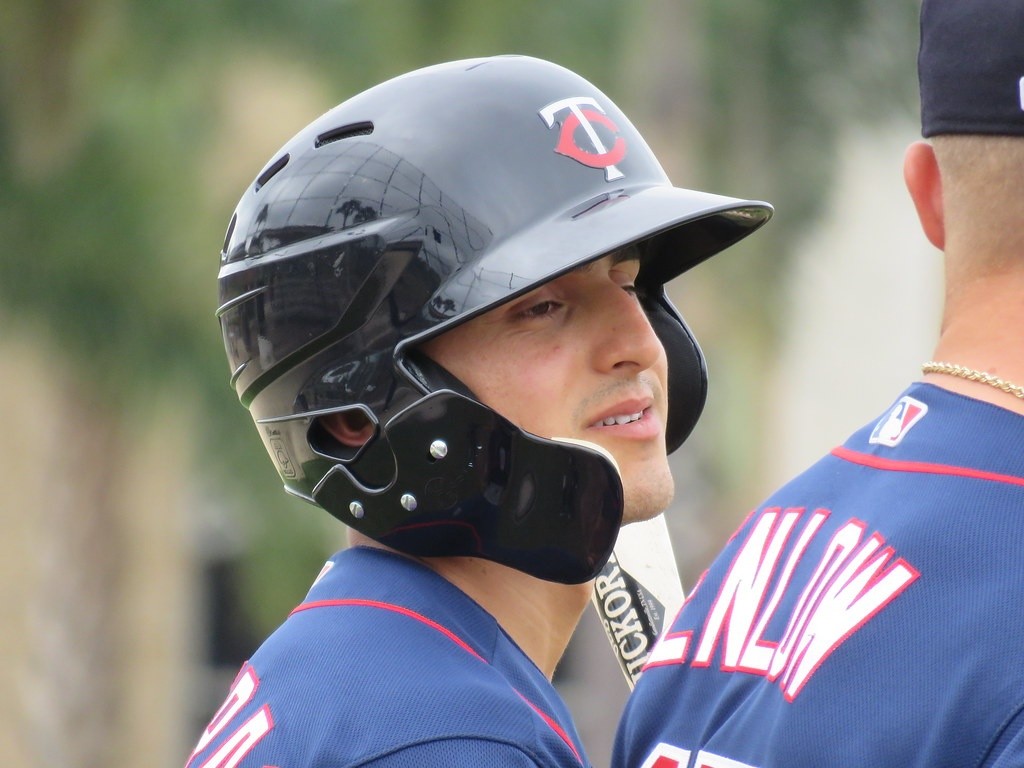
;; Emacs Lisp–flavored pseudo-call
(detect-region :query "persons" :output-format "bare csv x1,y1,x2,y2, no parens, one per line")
182,56,776,767
612,2,1024,767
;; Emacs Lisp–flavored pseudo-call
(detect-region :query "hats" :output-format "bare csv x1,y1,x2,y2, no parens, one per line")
918,0,1024,139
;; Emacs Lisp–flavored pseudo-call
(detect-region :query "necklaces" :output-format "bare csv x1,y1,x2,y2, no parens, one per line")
922,362,1024,399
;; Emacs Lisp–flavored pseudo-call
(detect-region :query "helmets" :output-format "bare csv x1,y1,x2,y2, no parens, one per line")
215,53,775,586
